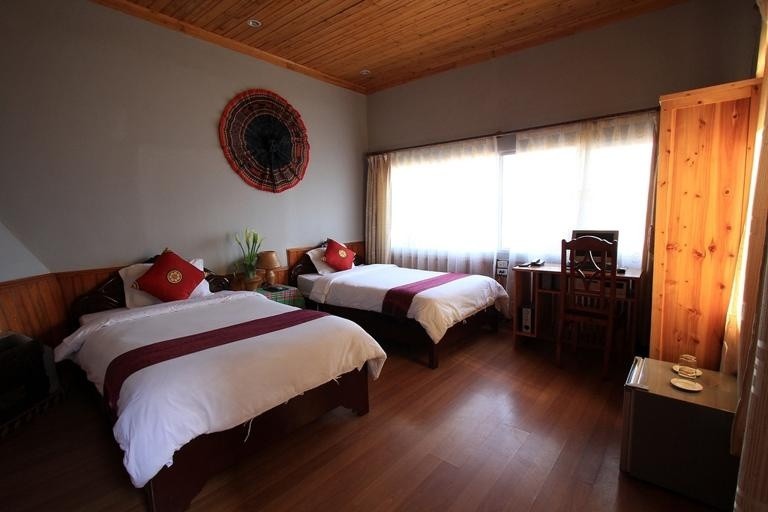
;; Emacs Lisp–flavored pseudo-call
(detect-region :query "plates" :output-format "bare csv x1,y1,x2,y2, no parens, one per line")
672,364,703,379
670,378,704,392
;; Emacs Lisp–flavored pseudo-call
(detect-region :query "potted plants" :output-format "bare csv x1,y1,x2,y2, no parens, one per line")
235,226,263,292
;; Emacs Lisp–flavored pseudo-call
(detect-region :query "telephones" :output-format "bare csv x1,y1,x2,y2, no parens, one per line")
531,259,544,266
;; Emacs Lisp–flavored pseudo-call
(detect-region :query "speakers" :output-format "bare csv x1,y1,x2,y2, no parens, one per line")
521,306,533,333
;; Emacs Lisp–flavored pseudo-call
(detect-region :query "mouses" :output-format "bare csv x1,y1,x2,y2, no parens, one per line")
617,268,626,273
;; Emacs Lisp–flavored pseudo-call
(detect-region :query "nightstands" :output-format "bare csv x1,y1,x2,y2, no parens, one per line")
256,284,298,307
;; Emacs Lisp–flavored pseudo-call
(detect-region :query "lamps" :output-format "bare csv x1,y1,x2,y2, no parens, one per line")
256,251,280,285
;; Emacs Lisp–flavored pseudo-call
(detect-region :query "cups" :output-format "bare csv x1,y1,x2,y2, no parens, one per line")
678,354,698,388
679,366,696,388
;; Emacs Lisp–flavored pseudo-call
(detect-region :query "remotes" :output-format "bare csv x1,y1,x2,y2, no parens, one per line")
270,286,282,290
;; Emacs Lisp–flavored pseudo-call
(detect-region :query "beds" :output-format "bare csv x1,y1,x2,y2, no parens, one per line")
54,255,389,511
286,241,513,369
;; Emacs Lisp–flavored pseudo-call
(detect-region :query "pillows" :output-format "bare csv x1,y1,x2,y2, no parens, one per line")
306,242,356,274
320,238,356,271
132,248,210,303
119,259,215,309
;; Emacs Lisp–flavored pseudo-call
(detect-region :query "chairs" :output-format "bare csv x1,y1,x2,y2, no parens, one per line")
555,236,618,381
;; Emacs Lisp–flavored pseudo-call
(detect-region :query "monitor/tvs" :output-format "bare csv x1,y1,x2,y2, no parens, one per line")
569,230,619,271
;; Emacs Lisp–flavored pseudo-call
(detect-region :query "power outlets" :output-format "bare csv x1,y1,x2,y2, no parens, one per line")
497,268,508,276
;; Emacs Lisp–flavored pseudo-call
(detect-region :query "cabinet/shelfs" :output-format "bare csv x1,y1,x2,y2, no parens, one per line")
620,356,743,506
512,261,642,358
0,331,65,440
